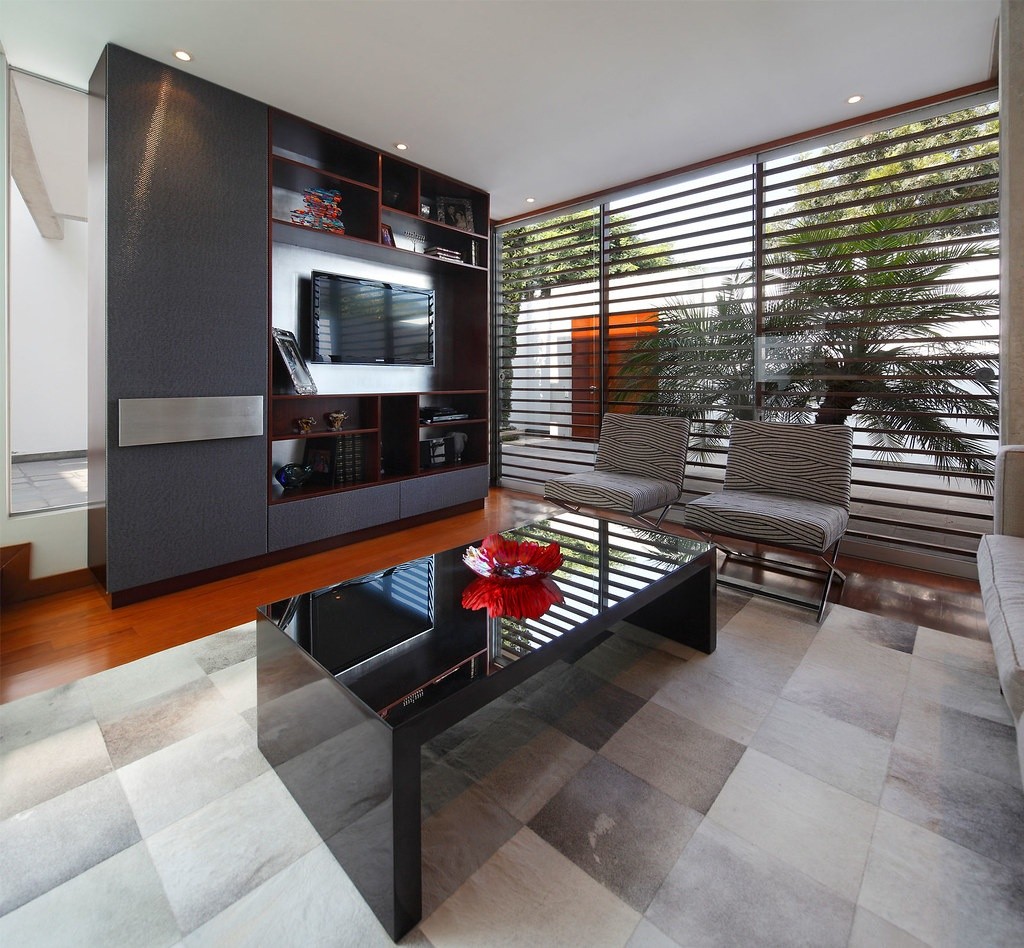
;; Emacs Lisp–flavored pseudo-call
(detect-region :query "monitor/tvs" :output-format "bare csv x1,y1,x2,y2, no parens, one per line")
309,270,435,368
310,555,433,676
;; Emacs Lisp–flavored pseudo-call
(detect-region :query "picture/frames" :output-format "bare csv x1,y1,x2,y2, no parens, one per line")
272,328,318,395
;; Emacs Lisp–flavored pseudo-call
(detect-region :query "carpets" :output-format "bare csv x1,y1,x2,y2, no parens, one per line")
0,576,1023,948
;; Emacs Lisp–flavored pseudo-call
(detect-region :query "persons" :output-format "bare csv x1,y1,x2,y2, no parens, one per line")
312,453,329,473
445,204,468,228
384,229,391,244
282,341,313,387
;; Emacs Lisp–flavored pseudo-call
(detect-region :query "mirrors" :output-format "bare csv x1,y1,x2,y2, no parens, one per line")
6,65,90,516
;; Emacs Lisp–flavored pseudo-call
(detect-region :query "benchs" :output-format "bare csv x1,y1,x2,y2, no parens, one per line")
977,444,1024,783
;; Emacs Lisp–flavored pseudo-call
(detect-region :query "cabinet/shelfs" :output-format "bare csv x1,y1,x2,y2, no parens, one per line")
89,42,491,610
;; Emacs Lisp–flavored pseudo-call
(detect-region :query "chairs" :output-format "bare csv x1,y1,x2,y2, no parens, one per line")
685,420,853,623
544,413,691,533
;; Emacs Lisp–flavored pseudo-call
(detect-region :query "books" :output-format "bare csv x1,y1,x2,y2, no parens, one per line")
425,246,464,262
458,238,486,265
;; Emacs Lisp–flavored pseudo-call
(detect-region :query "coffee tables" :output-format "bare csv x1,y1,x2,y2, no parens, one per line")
255,513,717,944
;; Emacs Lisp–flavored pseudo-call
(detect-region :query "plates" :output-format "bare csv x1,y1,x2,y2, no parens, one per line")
462,533,566,584
460,577,565,621
